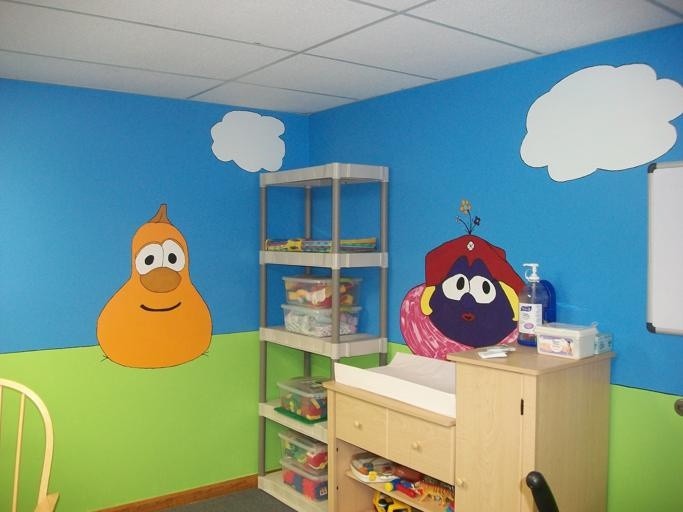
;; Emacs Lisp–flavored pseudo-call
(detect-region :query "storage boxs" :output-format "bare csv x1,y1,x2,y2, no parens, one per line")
280,275,363,307
279,302,362,338
275,376,329,421
277,428,327,476
277,458,327,503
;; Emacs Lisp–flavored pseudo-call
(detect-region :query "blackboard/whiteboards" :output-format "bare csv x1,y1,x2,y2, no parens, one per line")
646,161,683,335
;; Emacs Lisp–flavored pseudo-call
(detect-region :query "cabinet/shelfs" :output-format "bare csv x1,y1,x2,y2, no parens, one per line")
257,160,388,512
316,380,454,512
446,345,615,512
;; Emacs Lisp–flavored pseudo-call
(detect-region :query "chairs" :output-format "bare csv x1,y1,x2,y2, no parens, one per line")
0,378,60,512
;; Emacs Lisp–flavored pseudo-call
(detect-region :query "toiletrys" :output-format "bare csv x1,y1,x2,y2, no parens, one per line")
517,264,550,347
